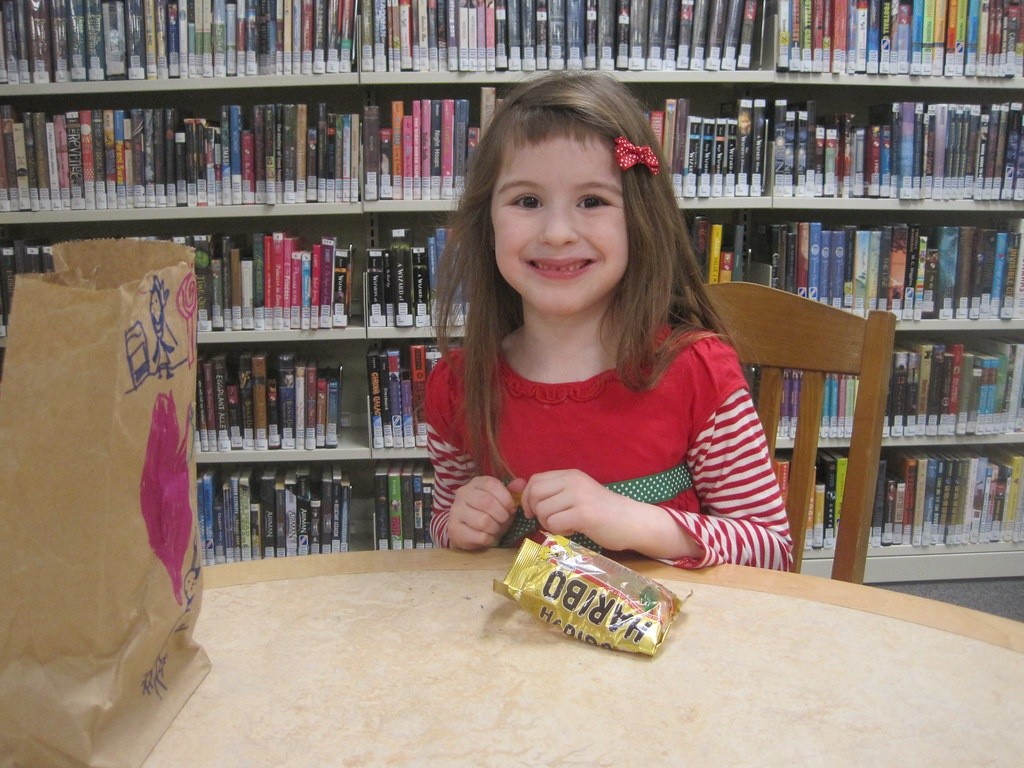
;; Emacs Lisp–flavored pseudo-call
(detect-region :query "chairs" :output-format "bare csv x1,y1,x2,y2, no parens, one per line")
688,281,897,584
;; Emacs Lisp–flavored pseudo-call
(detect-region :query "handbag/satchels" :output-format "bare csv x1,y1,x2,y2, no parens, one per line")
0,236,213,767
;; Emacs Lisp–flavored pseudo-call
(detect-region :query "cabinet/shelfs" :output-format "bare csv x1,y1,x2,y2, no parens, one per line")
0,0,1024,584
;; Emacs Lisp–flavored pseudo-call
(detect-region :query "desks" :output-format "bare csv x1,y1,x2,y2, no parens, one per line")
141,547,1024,768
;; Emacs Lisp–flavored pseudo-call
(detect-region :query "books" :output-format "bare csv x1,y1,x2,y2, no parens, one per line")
0,0,1024,568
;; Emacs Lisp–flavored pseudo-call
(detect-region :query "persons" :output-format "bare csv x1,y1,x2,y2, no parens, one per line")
429,76,795,573
